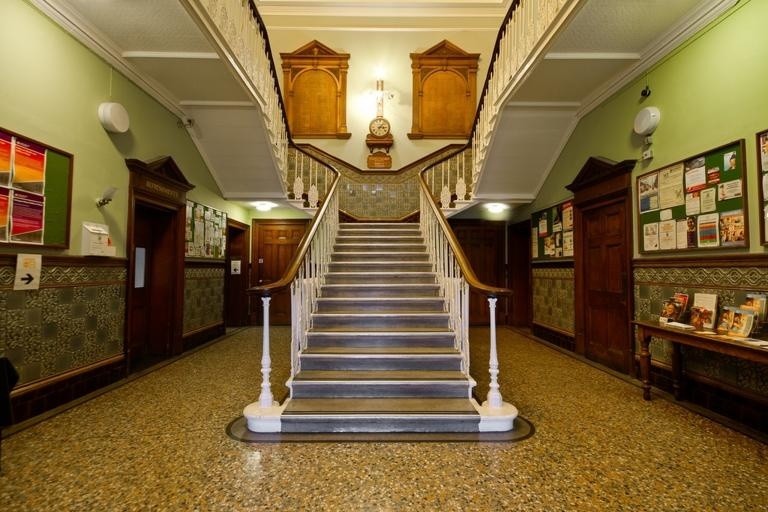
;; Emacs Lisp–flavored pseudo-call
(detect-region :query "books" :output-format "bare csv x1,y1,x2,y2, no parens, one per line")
660,293,768,348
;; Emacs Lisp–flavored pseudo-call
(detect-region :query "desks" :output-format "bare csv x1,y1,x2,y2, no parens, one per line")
629,319,768,401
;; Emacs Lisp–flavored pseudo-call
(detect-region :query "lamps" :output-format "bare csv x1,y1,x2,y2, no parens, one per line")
95,186,119,208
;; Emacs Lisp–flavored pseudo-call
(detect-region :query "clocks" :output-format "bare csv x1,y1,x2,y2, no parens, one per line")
369,117,390,138
